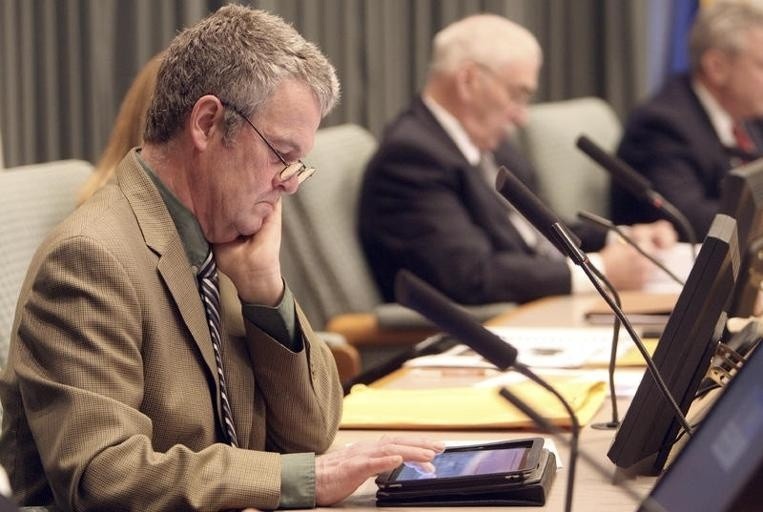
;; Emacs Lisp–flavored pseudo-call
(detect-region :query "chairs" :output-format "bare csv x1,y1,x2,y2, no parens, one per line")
278,123,389,331
494,96,627,228
0,156,98,382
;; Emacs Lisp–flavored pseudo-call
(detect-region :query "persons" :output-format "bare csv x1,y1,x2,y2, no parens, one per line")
0,2,445,510
66,36,372,395
356,12,679,301
608,0,763,248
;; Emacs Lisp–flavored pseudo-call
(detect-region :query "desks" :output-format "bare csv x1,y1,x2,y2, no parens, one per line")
240,244,761,512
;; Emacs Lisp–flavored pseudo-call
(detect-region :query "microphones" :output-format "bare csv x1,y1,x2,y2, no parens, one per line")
551,220,691,436
395,268,580,512
575,135,697,304
495,165,623,429
722,143,762,162
577,208,686,296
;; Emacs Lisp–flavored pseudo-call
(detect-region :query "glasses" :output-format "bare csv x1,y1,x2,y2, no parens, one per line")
476,64,534,104
218,102,316,186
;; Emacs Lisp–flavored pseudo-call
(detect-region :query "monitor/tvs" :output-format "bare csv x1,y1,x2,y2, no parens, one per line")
719,154,763,319
634,335,763,512
603,212,743,476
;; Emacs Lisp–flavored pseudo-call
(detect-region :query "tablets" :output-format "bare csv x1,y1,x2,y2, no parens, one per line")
375,434,545,491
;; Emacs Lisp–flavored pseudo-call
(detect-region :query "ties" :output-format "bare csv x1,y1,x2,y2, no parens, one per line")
195,243,239,447
732,122,760,164
